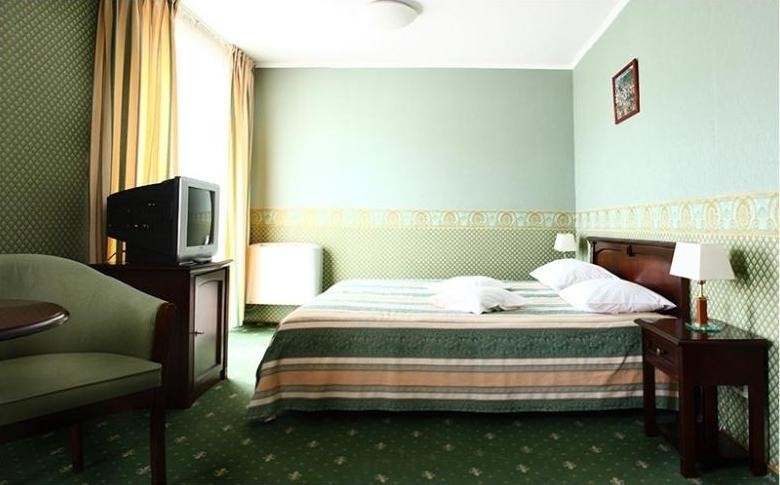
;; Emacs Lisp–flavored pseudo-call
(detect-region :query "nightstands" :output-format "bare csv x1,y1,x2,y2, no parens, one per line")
634,317,772,479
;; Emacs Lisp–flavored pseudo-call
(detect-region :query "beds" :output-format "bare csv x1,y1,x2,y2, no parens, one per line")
243,236,688,432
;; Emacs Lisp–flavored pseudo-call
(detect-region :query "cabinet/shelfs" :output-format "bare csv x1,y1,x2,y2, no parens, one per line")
113,258,230,408
244,243,324,326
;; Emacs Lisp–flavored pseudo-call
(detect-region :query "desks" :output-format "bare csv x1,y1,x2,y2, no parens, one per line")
0,299,70,342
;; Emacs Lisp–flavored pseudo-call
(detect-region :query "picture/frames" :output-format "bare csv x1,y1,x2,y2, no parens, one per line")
612,59,639,123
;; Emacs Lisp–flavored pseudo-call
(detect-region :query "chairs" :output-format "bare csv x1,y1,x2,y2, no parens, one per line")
0,252,178,484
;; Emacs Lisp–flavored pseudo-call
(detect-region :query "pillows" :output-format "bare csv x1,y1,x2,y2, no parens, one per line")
426,286,525,317
451,275,514,290
528,259,622,291
562,279,678,314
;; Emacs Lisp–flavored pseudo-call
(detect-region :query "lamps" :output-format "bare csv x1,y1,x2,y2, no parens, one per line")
365,0,418,31
553,233,578,259
668,242,736,332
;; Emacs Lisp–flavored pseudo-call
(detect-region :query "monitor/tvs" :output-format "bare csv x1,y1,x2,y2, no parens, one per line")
105,175,220,268
